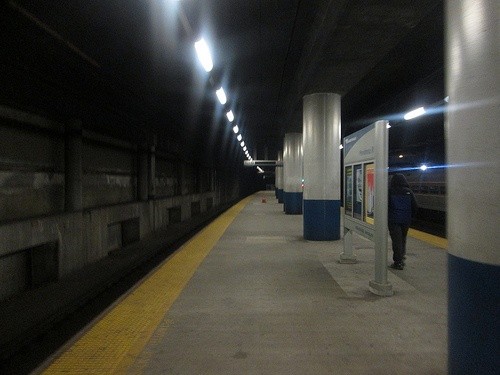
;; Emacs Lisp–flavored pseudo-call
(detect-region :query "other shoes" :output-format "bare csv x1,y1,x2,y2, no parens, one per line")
391,262,404,271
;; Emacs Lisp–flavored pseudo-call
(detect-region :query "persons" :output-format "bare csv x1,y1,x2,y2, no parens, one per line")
388,174,416,270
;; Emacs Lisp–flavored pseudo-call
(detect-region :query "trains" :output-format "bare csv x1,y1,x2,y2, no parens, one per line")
388,168,446,224
